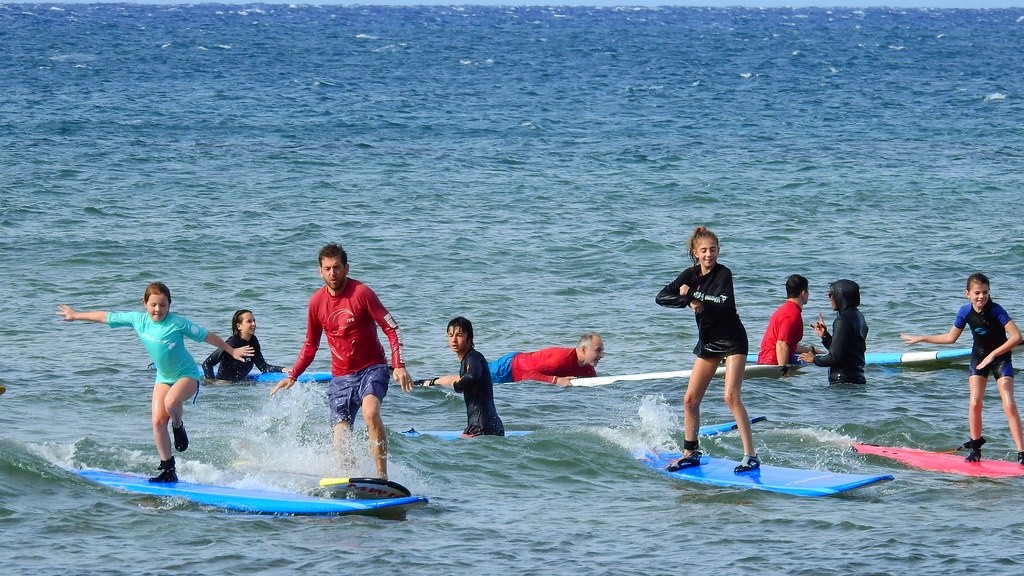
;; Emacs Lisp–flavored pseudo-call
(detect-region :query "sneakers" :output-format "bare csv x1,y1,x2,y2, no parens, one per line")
734,454,761,473
148,467,178,482
172,420,188,452
1018,452,1024,463
666,454,700,472
965,448,981,462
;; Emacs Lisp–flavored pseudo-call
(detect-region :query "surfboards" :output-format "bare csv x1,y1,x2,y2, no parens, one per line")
319,478,410,497
851,444,1024,478
747,349,974,365
642,452,895,496
400,431,532,438
76,467,429,514
570,365,787,386
147,363,332,384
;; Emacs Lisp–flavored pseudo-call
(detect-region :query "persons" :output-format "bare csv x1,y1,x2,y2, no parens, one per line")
756,274,829,376
900,272,1024,465
411,331,605,388
270,241,415,482
446,316,505,439
55,281,254,484
202,309,296,386
654,226,760,473
797,278,869,386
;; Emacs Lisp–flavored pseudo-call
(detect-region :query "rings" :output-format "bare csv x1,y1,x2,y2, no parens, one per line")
407,379,411,382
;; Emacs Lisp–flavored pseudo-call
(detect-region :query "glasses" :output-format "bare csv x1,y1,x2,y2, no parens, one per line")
828,293,832,299
